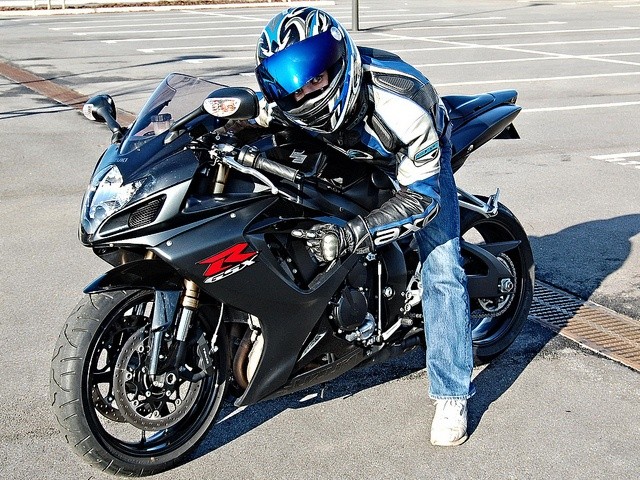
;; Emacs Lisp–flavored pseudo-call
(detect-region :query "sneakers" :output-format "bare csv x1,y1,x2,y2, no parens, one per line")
430,399,468,447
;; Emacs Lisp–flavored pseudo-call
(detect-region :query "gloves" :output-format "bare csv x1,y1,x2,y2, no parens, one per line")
290,214,375,266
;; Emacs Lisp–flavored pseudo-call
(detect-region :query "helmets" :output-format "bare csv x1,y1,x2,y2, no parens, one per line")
253,7,365,135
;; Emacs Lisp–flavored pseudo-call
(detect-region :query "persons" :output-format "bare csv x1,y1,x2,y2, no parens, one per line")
251,8,478,450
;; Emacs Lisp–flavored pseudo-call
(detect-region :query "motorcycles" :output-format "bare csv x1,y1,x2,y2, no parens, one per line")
50,74,535,476
